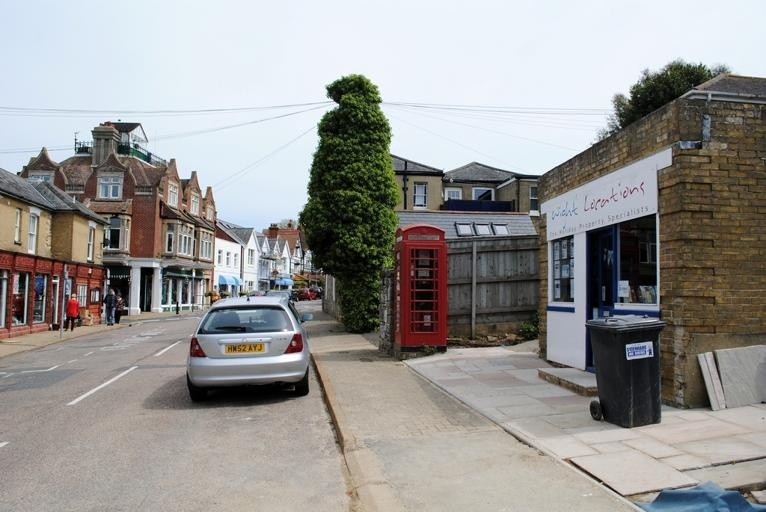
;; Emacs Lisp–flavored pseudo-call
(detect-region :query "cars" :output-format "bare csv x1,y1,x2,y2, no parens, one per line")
184,295,314,403
249,286,323,309
11,293,53,317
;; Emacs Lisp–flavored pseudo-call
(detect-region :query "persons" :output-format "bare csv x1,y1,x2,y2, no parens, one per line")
103,287,116,325
64,292,79,332
113,292,125,323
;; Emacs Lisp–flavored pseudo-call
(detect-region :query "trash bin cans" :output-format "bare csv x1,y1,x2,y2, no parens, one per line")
585,313,667,430
